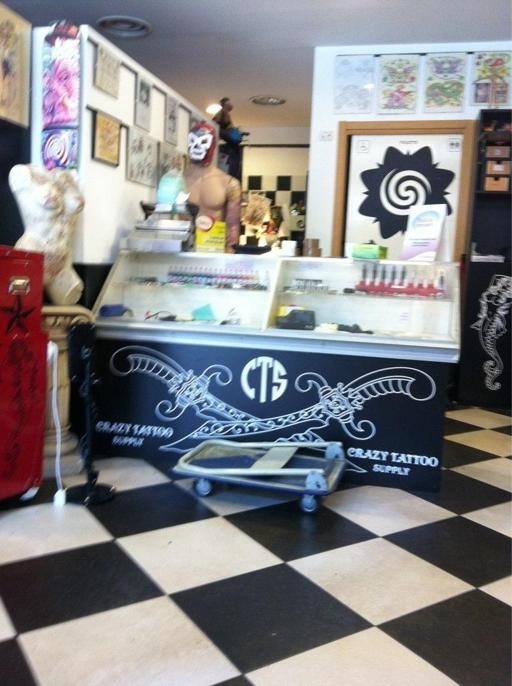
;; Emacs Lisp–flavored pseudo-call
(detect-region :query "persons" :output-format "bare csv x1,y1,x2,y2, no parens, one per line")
9,165,84,304
175,122,241,252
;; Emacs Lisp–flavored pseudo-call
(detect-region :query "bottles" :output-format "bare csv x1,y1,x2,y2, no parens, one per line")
171,263,261,290
100,304,127,317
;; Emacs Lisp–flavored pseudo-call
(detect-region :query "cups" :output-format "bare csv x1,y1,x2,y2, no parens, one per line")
281,239,297,258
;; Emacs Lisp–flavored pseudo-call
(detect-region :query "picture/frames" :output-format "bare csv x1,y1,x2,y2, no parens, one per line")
329,120,483,270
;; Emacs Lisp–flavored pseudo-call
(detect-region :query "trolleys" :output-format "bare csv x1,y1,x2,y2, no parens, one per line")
172,439,347,514
